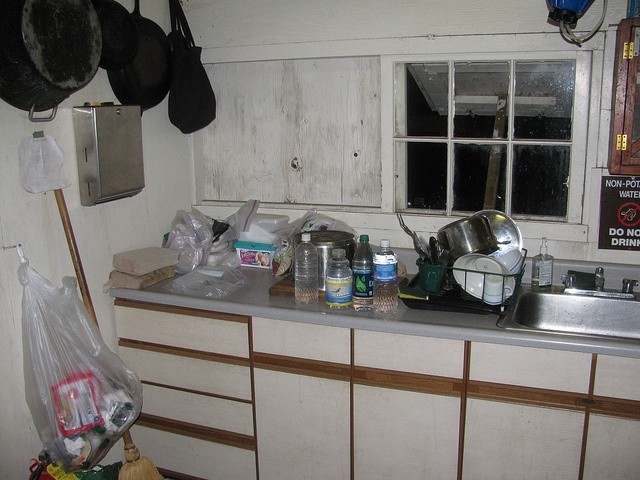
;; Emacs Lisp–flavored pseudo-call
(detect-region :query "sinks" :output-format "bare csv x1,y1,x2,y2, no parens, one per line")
498,288,640,361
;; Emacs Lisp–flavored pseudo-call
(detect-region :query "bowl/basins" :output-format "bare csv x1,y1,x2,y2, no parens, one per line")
474,210,523,253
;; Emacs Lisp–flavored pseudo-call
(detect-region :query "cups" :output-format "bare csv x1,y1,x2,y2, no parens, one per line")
415,259,447,292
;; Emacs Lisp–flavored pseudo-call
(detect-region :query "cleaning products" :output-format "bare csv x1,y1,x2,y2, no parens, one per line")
532,237,552,287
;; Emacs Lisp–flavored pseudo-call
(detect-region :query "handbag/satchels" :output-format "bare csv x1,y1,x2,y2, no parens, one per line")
161,1,216,134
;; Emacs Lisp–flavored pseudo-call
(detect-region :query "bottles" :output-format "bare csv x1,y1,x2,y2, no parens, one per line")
373,239,400,313
324,248,353,306
351,235,374,312
294,234,319,302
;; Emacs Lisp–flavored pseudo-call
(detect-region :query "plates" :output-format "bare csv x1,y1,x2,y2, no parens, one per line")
499,250,522,274
452,254,515,305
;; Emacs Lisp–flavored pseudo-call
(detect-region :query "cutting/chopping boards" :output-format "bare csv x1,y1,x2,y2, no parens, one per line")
269,273,408,301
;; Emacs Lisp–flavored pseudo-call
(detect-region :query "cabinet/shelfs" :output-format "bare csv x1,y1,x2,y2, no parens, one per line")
460,341,594,479
112,298,257,480
580,352,640,480
349,326,470,480
248,315,353,478
607,17,640,176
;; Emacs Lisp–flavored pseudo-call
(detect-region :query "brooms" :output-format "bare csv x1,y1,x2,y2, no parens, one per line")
54,188,163,480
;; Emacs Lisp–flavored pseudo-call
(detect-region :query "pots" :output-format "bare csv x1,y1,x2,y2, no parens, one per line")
21,1,102,90
0,1,78,121
107,1,173,116
93,1,139,71
164,32,187,57
437,215,500,261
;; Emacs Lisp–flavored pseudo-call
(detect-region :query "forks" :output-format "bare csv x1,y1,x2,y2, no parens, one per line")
396,215,450,264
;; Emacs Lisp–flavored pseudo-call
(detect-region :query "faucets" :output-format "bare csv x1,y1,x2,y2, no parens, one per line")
593,267,605,290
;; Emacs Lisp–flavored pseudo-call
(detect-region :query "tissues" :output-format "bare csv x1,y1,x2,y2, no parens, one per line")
234,226,275,270
